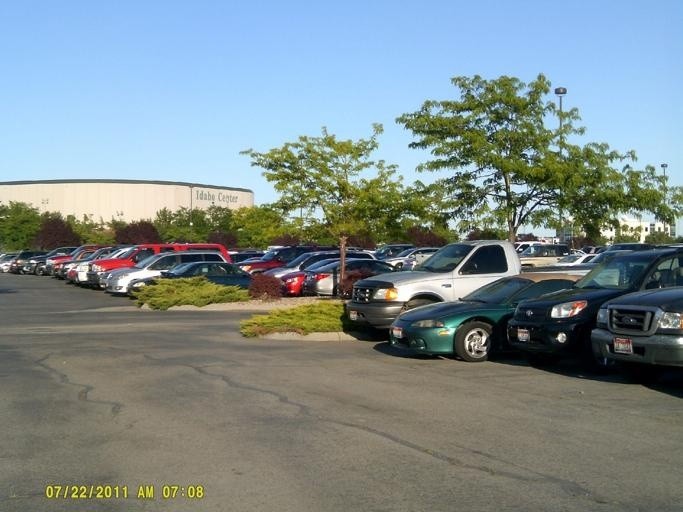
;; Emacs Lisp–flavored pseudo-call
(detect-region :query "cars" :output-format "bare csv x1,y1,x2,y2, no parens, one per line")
510,239,682,285
386,274,595,365
0,240,440,297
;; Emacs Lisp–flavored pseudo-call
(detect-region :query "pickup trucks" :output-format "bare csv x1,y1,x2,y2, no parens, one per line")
340,236,626,338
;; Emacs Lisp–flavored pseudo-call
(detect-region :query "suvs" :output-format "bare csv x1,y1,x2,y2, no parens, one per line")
503,241,682,368
590,283,682,365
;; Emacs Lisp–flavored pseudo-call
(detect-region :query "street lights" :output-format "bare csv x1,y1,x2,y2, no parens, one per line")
188,183,193,228
659,163,667,233
554,86,568,234
